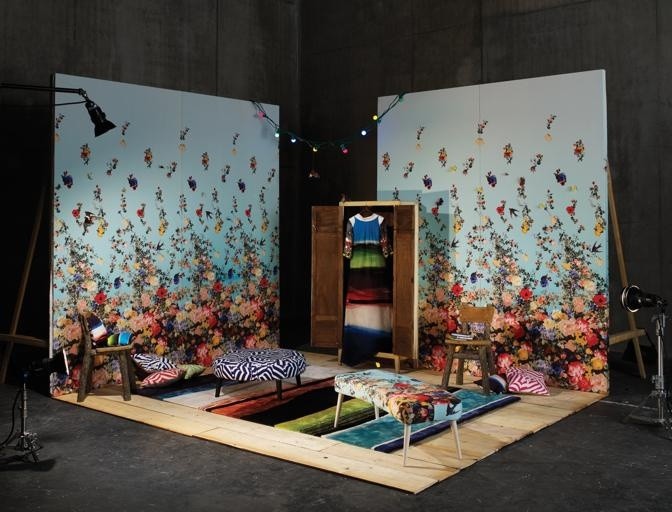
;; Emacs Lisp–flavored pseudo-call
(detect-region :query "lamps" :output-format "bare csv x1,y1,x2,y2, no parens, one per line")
619,282,672,433
1,349,74,469
1,77,117,139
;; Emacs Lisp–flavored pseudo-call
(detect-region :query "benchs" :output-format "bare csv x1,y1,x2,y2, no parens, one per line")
331,369,464,467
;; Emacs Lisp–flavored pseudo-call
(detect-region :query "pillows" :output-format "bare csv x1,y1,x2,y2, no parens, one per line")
507,368,550,396
83,313,114,347
130,351,177,372
99,330,135,348
177,363,205,379
139,368,188,388
474,372,507,394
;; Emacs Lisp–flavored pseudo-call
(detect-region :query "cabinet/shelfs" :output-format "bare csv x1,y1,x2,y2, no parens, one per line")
309,199,420,371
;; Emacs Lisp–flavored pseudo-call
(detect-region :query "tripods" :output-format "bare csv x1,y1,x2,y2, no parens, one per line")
622,315,672,431
3,387,46,471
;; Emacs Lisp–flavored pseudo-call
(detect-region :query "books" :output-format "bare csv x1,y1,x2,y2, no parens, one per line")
452,331,472,343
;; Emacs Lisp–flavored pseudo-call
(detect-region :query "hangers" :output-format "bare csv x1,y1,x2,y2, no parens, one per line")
358,202,373,217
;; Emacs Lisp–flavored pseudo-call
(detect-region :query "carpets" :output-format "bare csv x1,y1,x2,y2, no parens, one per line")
130,360,521,454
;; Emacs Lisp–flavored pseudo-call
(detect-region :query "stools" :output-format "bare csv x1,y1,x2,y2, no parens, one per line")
212,347,307,399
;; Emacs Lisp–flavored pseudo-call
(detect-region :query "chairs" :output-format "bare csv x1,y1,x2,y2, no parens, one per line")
76,311,137,403
442,305,498,395
